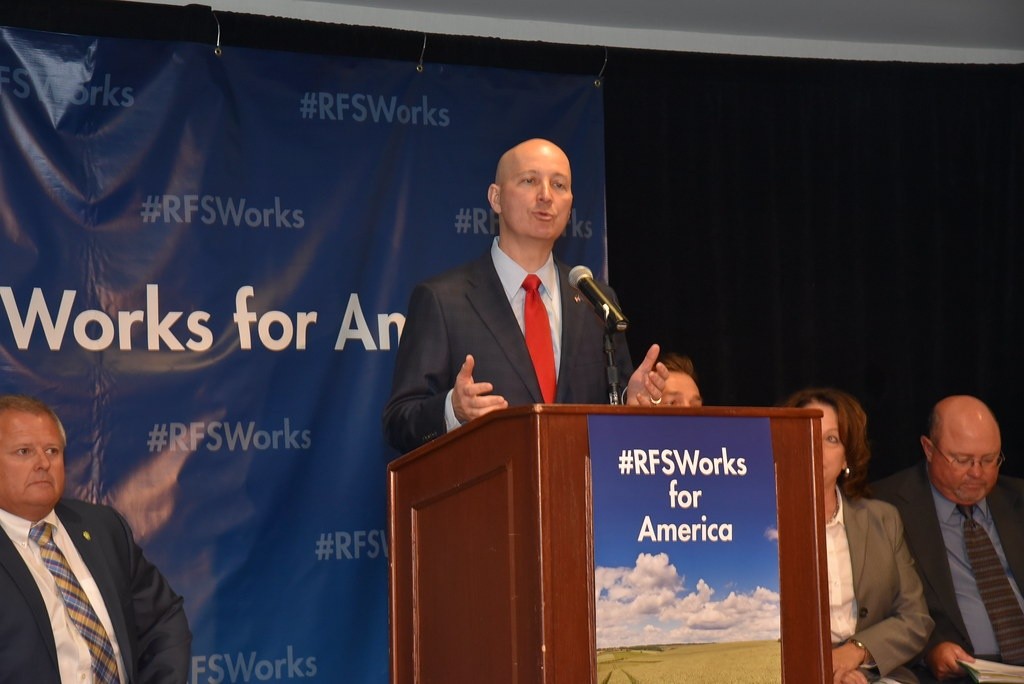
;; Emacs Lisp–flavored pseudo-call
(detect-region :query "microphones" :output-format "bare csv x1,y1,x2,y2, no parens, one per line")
568,265,629,332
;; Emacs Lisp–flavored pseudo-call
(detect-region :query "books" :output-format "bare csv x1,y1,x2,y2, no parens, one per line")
955,658,1024,684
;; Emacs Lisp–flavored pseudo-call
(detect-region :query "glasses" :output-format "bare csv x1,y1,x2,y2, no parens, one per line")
930,438,1005,473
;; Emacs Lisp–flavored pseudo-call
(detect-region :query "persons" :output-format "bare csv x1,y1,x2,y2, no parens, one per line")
656,352,702,407
384,138,670,457
866,394,1024,684
784,389,936,683
0,391,194,684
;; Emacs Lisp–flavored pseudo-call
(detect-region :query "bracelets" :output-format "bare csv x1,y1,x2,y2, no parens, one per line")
851,639,866,650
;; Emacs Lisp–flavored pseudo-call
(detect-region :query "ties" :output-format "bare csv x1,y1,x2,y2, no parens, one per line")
955,503,1024,666
521,273,555,405
29,522,120,684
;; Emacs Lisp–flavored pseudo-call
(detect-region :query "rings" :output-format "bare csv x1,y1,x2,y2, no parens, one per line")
651,397,662,405
